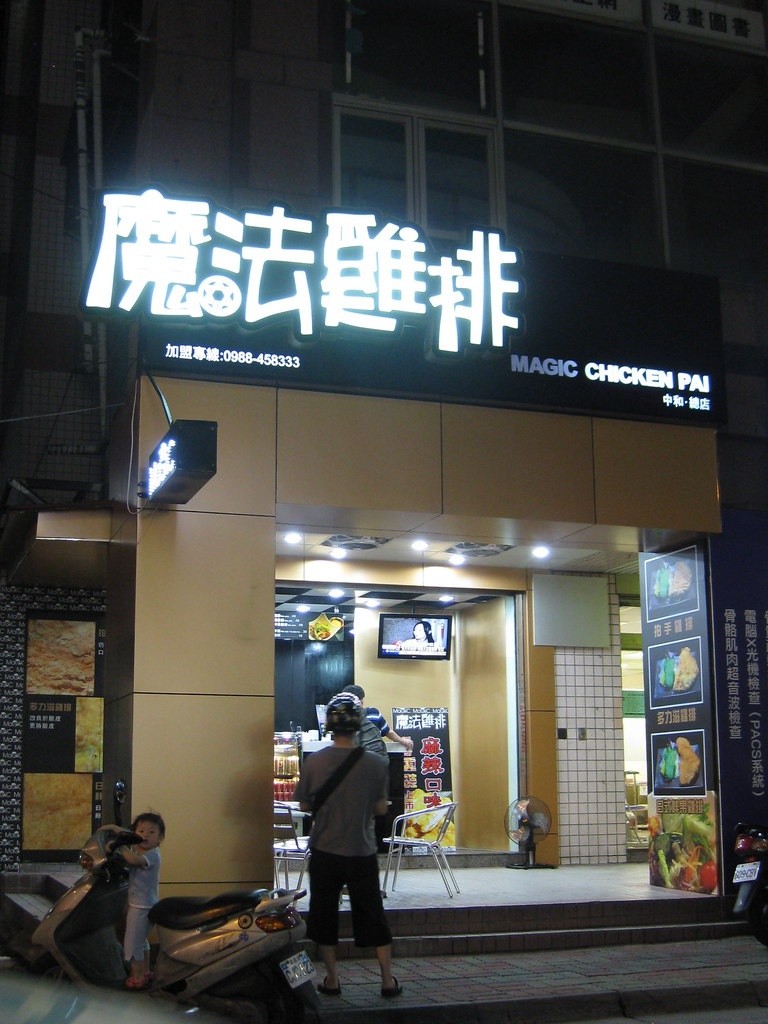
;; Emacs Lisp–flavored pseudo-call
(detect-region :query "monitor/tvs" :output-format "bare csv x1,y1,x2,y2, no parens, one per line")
378,613,453,660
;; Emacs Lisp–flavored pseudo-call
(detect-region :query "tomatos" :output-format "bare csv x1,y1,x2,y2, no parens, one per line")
699,858,718,890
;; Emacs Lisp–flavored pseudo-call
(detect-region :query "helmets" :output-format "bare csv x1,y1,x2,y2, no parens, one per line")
324,692,364,731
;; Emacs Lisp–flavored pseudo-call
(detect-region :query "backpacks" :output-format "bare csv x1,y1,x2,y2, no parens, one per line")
355,708,390,767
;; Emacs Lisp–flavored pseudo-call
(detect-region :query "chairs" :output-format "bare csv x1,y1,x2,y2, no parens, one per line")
273,800,312,908
382,803,460,899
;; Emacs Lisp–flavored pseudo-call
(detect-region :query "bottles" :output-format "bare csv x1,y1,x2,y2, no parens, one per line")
274,739,298,774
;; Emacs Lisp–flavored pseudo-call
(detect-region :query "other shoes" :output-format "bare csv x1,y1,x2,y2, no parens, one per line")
125,972,153,988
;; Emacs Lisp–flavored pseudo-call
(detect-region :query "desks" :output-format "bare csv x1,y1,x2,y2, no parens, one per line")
274,802,388,899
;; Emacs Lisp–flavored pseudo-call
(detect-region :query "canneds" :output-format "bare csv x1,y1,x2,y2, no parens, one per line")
274,755,298,776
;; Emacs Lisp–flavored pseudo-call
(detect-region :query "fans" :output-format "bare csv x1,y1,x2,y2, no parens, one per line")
503,796,558,870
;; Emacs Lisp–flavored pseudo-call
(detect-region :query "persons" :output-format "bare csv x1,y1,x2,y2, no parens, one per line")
96,813,165,989
344,685,414,751
297,693,402,997
401,621,442,651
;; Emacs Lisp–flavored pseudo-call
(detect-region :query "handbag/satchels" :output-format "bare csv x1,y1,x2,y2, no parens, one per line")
302,814,316,836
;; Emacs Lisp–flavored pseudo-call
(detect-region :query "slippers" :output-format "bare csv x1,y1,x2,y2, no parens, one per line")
317,975,342,996
381,975,403,996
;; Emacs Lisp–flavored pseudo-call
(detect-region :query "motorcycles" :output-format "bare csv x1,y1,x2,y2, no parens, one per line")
19,823,321,1023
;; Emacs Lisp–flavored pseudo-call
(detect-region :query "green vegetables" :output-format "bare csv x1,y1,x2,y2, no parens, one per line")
649,802,714,889
